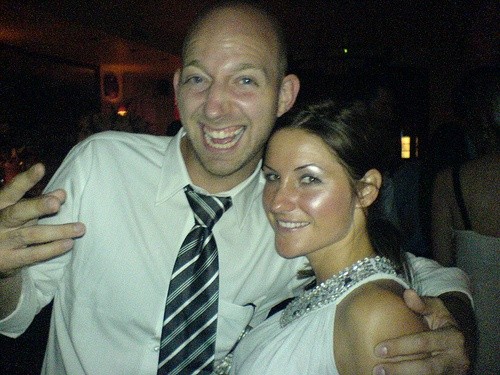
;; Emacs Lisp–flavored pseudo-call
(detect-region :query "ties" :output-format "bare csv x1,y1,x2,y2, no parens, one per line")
156,183,234,375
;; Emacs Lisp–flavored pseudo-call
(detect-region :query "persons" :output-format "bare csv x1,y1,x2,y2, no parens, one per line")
0,0,479,374
206,98,434,374
436,80,500,375
0,80,177,199
